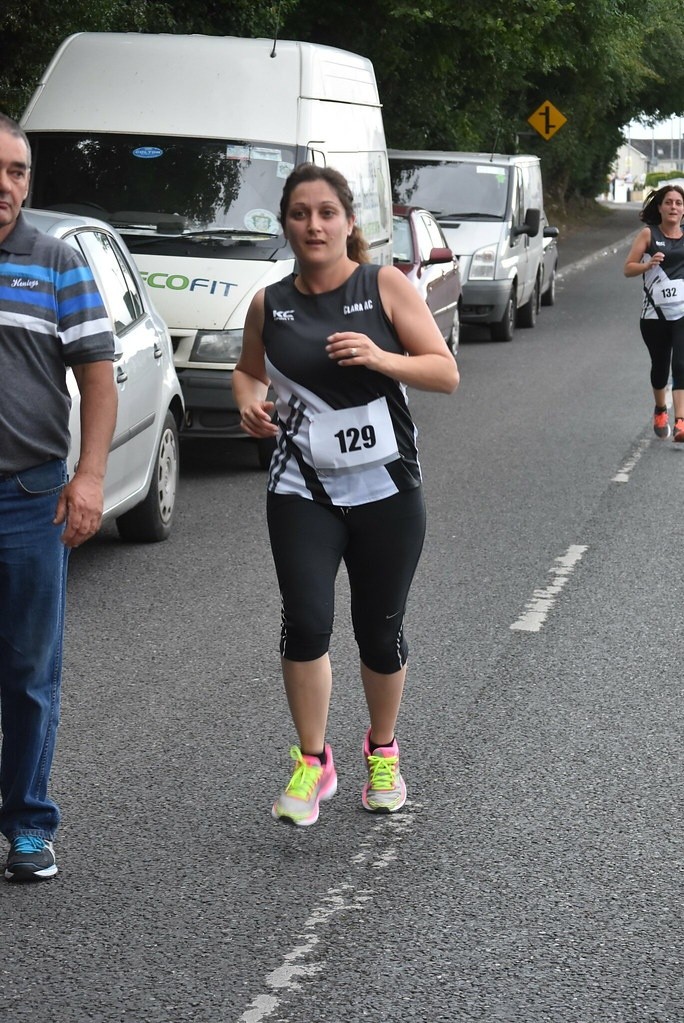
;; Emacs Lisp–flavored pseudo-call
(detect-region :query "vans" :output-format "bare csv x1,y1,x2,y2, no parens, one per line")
386,148,544,340
20,31,398,466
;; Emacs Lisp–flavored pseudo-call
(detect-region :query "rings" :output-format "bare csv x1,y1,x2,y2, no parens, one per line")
351,348,356,358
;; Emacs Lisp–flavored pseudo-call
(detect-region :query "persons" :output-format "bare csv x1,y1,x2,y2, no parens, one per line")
0,113,117,882
231,164,461,828
623,187,684,442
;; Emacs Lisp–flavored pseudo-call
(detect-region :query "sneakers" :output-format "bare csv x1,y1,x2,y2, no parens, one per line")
672,418,684,443
360,725,407,813
4,836,59,885
270,742,338,827
653,404,671,439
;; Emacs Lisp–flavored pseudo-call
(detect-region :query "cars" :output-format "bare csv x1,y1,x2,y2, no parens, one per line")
19,208,186,542
393,204,465,356
541,209,560,311
